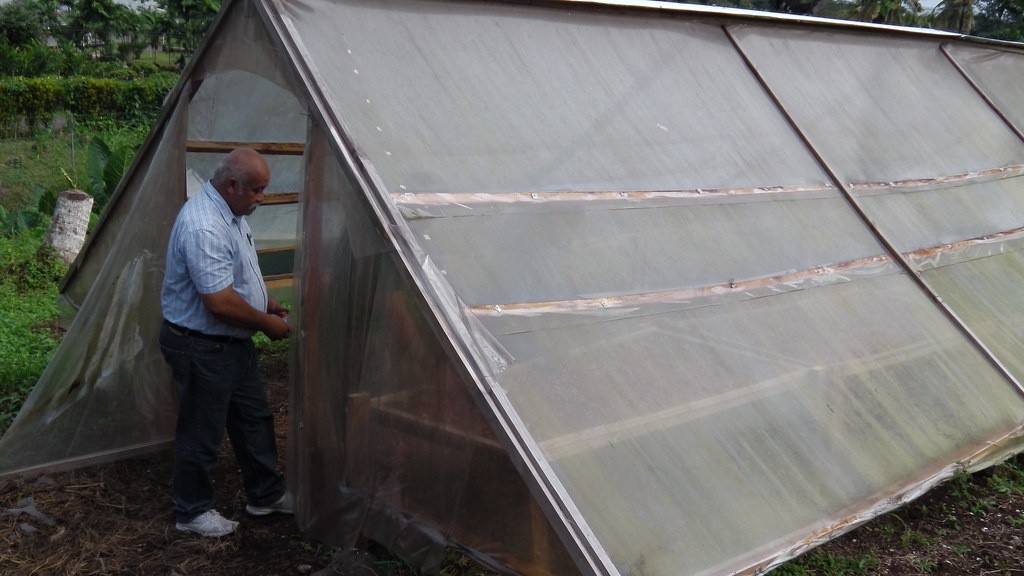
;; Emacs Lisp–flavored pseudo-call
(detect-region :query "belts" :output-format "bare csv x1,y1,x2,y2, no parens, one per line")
164,318,251,347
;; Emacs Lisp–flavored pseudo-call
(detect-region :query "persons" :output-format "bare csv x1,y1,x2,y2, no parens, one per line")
158,149,293,537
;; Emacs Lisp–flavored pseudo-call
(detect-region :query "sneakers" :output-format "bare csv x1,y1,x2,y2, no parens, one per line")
246,490,293,515
175,509,239,538
